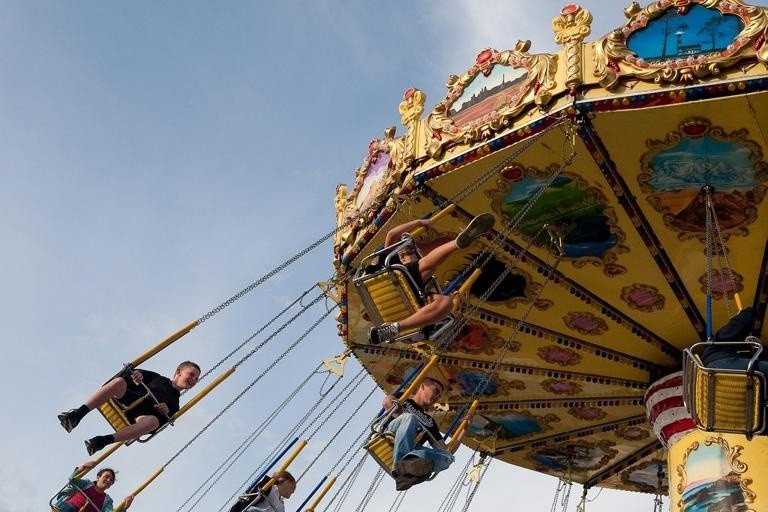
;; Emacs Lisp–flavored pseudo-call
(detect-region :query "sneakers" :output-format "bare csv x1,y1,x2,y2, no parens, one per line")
368,322,400,347
455,212,495,250
85,435,105,456
57,409,80,433
394,457,434,492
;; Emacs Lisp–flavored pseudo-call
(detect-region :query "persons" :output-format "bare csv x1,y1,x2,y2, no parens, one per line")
54,460,134,511
55,359,200,456
696,305,768,434
371,376,474,492
362,212,496,344
229,469,297,512
